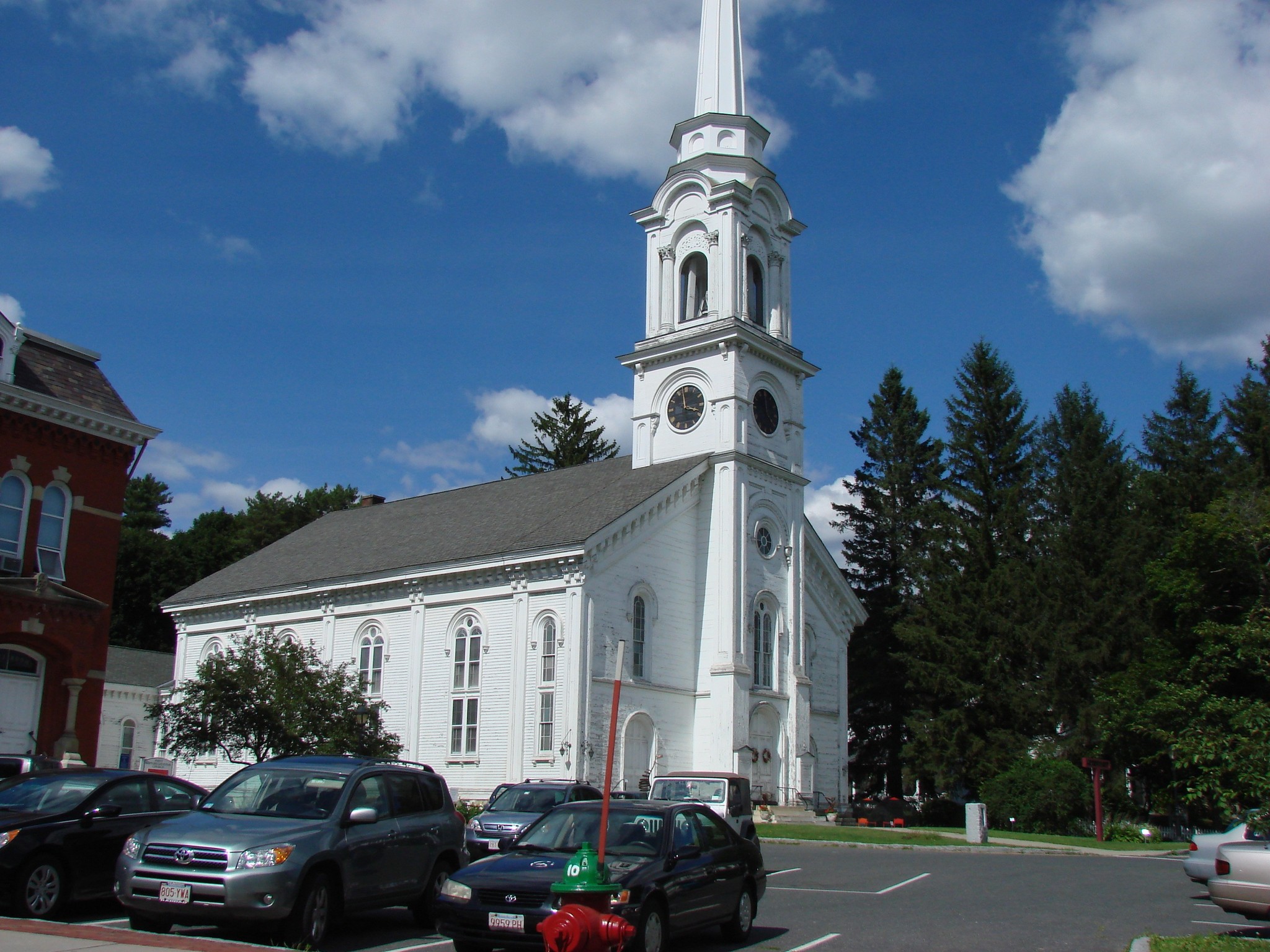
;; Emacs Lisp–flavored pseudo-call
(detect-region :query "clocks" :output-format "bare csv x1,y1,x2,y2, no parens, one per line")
752,388,780,437
665,383,707,433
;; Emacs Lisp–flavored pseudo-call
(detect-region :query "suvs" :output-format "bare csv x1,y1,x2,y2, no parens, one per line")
466,778,603,861
113,753,468,952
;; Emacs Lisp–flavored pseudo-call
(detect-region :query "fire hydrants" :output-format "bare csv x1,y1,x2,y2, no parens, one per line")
537,841,636,952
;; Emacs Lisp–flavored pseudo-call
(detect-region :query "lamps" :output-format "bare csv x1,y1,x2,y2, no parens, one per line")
559,741,571,756
581,740,594,758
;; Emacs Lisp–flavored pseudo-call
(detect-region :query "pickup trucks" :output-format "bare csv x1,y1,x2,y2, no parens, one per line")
0,754,97,804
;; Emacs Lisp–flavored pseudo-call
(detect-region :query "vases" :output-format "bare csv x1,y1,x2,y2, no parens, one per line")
760,810,769,820
826,813,837,822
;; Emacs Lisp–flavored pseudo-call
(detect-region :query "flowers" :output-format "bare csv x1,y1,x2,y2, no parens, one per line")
824,807,838,815
759,805,768,810
751,748,771,763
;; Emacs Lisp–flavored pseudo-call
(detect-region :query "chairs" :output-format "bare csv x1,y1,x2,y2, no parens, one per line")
858,818,876,827
883,818,903,828
617,820,715,853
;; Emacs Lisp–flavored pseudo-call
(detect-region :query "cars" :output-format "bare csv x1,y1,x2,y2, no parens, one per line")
1205,842,1270,924
634,770,762,857
1183,808,1270,887
0,767,234,920
436,799,767,952
